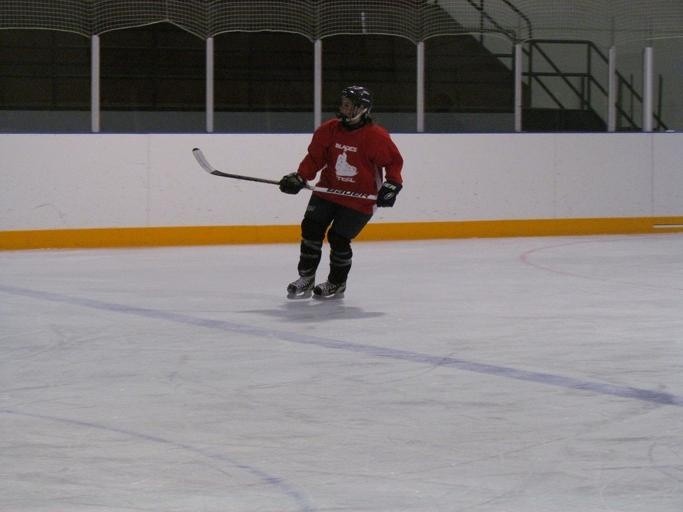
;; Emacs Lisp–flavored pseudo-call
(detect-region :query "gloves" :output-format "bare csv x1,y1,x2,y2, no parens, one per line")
279,172,305,194
377,180,403,208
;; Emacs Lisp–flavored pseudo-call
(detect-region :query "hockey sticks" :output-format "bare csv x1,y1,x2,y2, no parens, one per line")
192,148,379,200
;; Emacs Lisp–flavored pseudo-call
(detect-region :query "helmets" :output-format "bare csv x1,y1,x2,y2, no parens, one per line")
341,86,373,114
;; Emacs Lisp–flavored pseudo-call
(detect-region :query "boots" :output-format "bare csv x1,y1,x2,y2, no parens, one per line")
287,275,346,297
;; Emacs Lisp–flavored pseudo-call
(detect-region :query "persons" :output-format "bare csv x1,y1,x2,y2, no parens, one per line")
279,85,403,297
